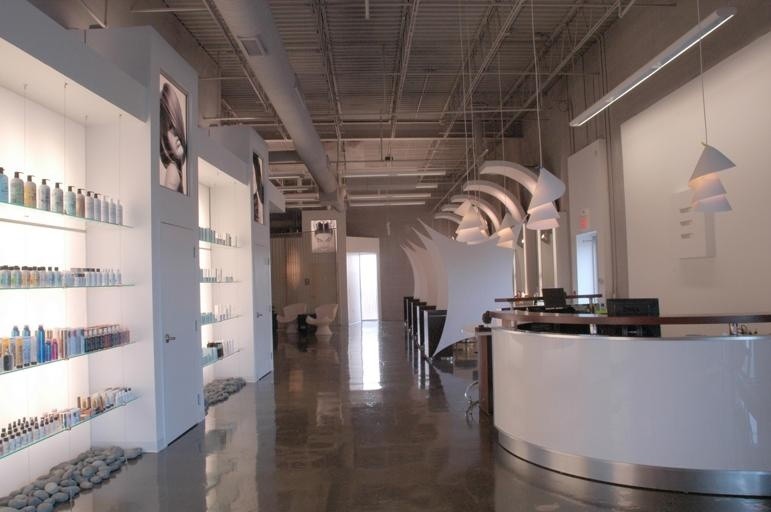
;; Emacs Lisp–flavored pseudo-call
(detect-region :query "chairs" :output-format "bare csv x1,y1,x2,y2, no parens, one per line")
304,302,341,340
273,300,310,336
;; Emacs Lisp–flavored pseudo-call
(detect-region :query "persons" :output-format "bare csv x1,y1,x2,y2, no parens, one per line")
312,222,334,252
159,82,185,194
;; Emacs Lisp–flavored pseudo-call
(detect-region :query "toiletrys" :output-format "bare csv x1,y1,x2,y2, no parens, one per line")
0,167,135,374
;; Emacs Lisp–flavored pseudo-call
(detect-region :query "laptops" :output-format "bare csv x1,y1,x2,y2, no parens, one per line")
541,287,569,311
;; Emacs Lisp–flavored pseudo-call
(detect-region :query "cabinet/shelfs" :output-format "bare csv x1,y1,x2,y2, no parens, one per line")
0,196,141,463
198,235,243,369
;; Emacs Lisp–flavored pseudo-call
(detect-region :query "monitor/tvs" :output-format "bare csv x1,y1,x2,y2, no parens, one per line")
606,298,661,338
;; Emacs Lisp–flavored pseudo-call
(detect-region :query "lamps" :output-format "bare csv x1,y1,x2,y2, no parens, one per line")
568,2,740,134
682,1,740,219
450,0,567,254
